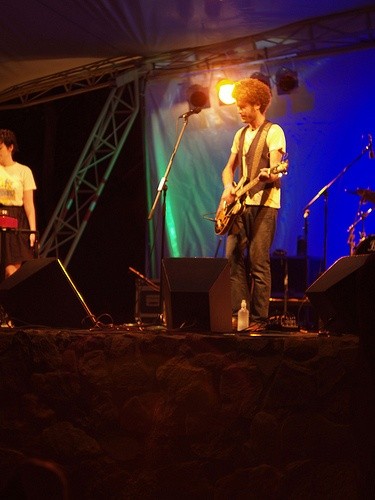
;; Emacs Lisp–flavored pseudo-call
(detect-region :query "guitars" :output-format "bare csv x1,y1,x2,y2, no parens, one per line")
215,161,289,236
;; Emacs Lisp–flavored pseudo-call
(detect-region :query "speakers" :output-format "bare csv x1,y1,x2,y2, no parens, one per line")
0,257,99,330
161,256,234,333
305,252,375,335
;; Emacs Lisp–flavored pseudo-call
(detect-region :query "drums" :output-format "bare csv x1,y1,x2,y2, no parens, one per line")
353,234,375,254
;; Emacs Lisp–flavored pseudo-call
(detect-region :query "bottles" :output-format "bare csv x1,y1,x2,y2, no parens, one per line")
237,299,249,331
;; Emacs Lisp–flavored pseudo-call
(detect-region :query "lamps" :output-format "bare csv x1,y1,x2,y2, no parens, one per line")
186,58,298,112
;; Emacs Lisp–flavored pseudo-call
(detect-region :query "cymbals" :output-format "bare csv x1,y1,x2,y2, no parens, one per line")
346,187,375,203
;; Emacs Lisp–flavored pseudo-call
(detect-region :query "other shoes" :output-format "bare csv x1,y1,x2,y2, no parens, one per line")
246,321,266,331
232,316,238,330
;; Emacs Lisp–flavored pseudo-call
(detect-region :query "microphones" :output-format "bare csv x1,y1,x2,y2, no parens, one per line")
178,106,201,118
368,133,375,158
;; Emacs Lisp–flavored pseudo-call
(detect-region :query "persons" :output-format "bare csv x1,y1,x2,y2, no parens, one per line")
1,129,36,328
0,448,68,500
222,79,286,330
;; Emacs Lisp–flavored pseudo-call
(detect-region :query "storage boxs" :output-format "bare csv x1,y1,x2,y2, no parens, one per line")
136,279,161,325
